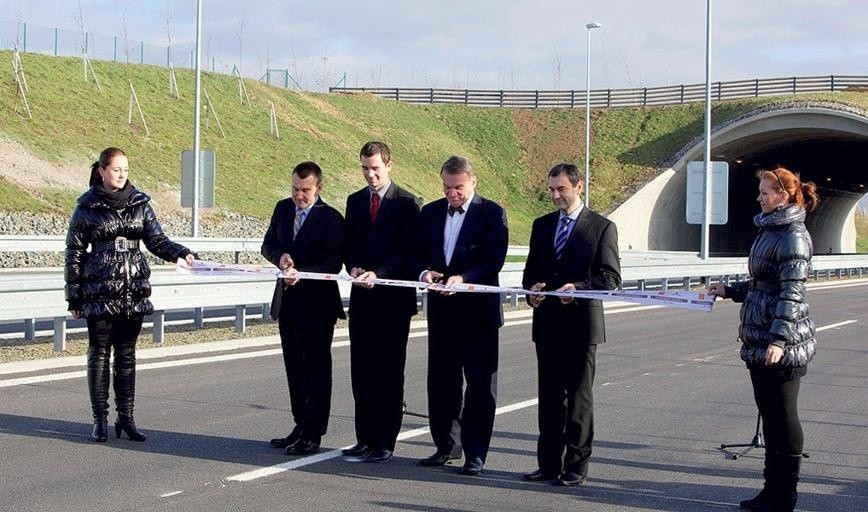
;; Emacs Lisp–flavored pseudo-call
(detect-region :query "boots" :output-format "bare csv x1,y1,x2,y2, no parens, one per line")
88,349,110,442
740,454,801,512
113,351,146,441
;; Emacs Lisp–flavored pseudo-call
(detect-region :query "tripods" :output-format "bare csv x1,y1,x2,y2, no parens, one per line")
721,412,810,460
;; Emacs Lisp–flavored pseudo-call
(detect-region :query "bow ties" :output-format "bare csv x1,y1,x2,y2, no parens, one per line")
448,205,465,217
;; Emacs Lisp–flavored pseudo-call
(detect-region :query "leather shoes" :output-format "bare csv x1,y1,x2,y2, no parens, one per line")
342,444,368,455
527,469,546,481
287,439,319,454
271,437,290,448
558,472,586,486
423,452,462,466
464,457,483,474
369,449,392,462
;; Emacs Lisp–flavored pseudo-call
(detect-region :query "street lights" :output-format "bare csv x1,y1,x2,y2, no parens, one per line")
583,21,603,209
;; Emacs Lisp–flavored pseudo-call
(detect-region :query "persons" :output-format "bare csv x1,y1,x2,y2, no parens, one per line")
409,157,508,475
522,163,622,486
707,167,821,512
65,146,195,443
342,141,420,463
261,162,345,455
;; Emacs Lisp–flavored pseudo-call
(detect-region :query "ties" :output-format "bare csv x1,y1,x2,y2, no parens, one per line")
371,192,379,222
554,217,572,260
294,211,306,232
